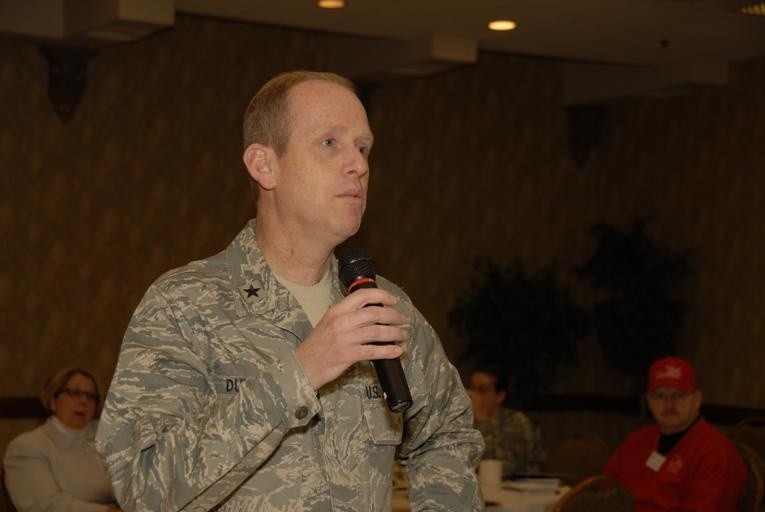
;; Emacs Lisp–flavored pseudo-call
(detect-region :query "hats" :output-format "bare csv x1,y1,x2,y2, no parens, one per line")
646,358,696,393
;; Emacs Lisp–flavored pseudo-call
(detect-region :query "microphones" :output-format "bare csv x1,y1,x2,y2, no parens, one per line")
338,251,413,414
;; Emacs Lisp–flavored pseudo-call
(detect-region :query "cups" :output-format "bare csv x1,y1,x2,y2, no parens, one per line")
479,457,505,486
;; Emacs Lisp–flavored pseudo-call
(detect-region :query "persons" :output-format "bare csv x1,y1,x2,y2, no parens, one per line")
94,71,485,512
3,367,122,512
466,366,546,479
598,356,748,512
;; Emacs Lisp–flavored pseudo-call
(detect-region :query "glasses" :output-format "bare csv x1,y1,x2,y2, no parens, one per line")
66,388,97,400
